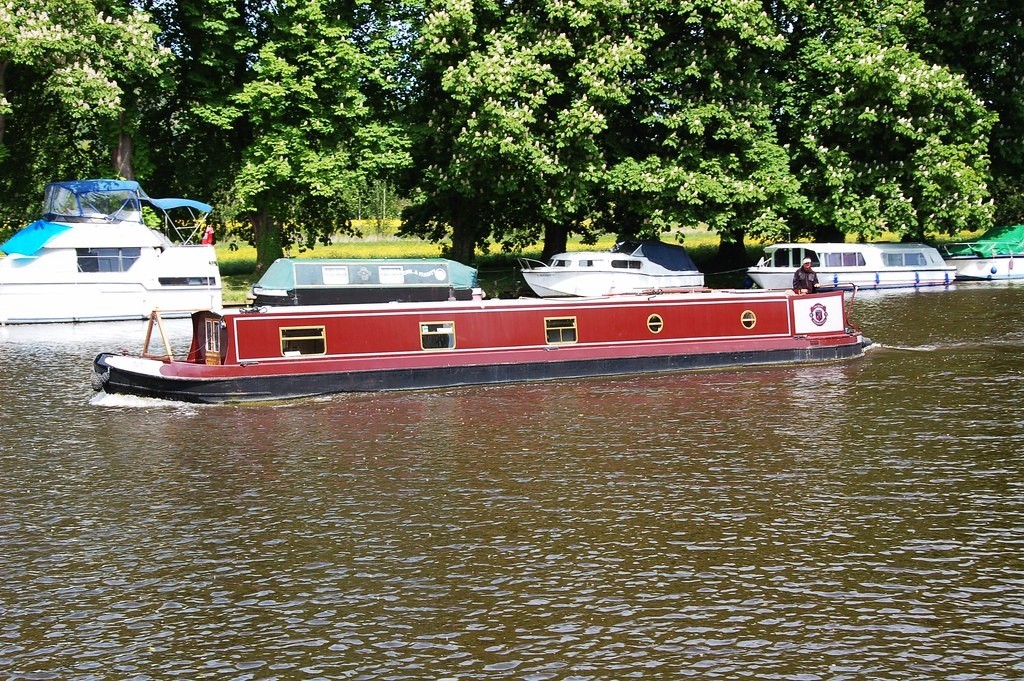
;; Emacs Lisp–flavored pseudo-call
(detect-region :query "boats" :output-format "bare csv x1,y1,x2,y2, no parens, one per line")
746,241,959,291
942,223,1024,281
0,178,225,327
90,258,875,406
517,240,705,299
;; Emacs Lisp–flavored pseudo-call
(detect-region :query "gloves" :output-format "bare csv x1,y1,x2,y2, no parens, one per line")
801,289,808,295
814,284,820,288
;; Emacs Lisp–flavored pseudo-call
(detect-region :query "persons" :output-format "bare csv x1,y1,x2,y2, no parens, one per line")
793,258,821,294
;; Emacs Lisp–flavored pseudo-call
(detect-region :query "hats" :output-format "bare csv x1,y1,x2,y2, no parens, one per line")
802,258,811,265
